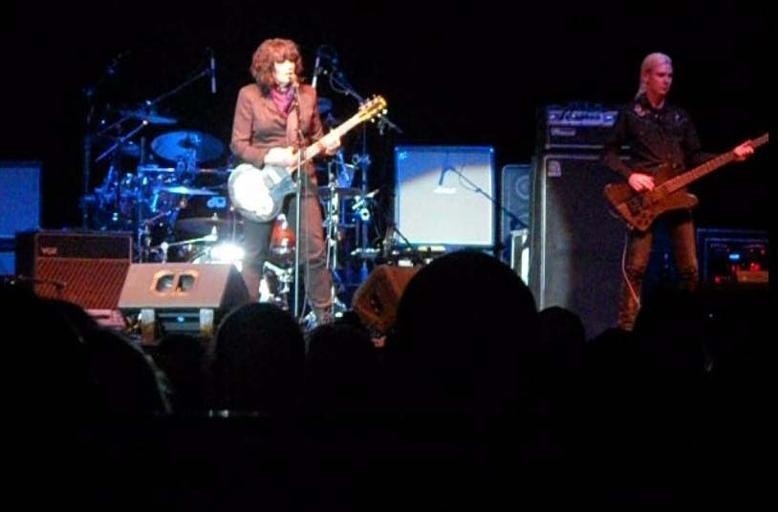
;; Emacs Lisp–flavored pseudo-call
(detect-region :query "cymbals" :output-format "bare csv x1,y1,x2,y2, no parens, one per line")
151,132,222,164
120,112,177,124
318,186,359,201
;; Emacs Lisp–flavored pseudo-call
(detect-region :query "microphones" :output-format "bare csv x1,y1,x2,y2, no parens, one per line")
289,72,299,87
311,56,320,88
209,58,217,95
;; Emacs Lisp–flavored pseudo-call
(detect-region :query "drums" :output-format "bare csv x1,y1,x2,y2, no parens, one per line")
138,169,228,220
151,186,220,218
269,214,297,254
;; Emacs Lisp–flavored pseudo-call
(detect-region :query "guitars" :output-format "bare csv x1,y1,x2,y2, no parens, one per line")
603,135,768,231
228,95,388,224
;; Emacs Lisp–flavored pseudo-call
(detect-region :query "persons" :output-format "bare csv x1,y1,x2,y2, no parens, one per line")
595,52,755,331
2,248,777,512
229,36,342,326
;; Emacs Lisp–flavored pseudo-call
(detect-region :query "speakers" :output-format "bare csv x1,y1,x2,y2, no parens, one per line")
499,164,531,267
15,233,133,309
117,262,249,353
